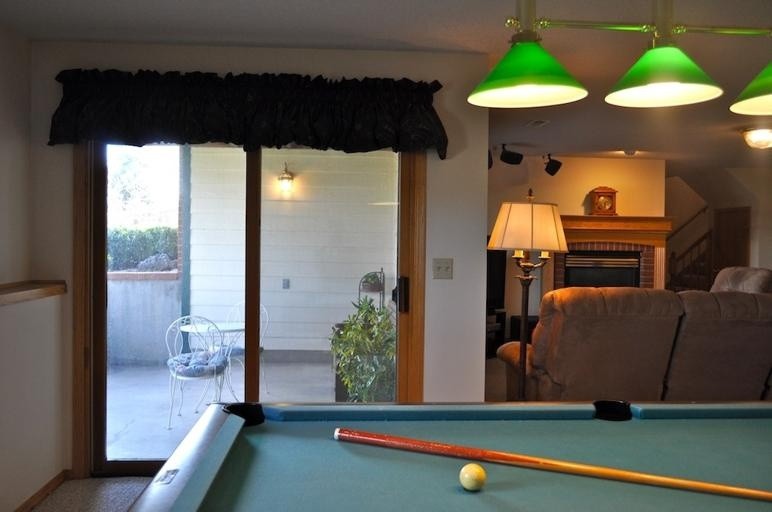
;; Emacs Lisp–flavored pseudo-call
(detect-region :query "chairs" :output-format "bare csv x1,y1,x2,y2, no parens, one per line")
495,265,770,403
164,301,270,431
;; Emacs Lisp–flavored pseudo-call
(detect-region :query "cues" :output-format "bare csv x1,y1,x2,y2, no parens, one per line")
334,428,771,499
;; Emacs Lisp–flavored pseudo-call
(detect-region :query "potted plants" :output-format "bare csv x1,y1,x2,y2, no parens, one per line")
362,271,381,290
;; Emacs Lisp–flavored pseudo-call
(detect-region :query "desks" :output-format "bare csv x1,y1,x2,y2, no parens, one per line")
121,400,771,510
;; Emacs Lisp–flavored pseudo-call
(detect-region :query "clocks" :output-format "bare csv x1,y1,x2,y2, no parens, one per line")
588,186,619,216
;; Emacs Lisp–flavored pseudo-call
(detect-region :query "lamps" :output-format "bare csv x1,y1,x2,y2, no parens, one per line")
486,189,570,401
742,125,772,150
729,1,772,115
543,152,563,175
466,0,590,109
499,145,522,166
605,1,724,108
487,150,494,169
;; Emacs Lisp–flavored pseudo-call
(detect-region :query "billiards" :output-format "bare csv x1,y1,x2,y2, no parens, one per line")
459,462,486,492
